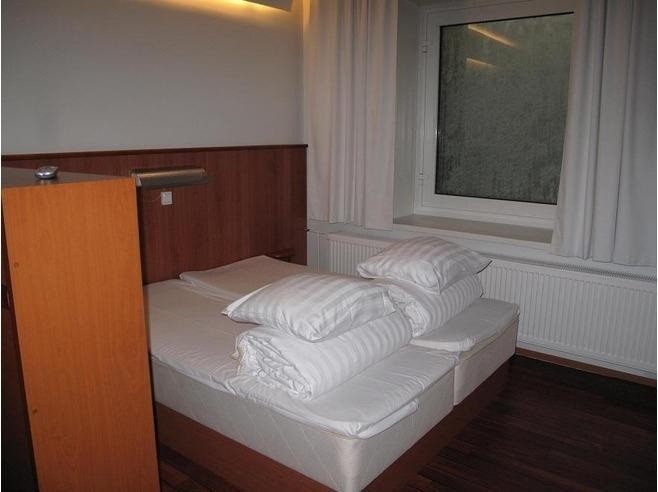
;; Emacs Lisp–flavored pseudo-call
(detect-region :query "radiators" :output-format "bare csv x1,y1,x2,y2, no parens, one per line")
326,233,657,388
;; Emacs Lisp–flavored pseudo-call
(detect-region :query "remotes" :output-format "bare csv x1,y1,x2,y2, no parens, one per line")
35,165,59,178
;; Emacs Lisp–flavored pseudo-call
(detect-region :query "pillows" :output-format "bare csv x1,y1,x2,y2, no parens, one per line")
219,268,393,342
356,232,492,292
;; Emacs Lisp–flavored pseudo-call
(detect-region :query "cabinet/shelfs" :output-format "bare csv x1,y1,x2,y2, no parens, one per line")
1,167,165,491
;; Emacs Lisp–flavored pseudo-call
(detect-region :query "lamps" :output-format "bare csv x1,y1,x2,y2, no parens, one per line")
129,164,209,188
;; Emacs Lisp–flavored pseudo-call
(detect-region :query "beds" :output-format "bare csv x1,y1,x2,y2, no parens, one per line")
139,278,455,492
177,253,521,406
